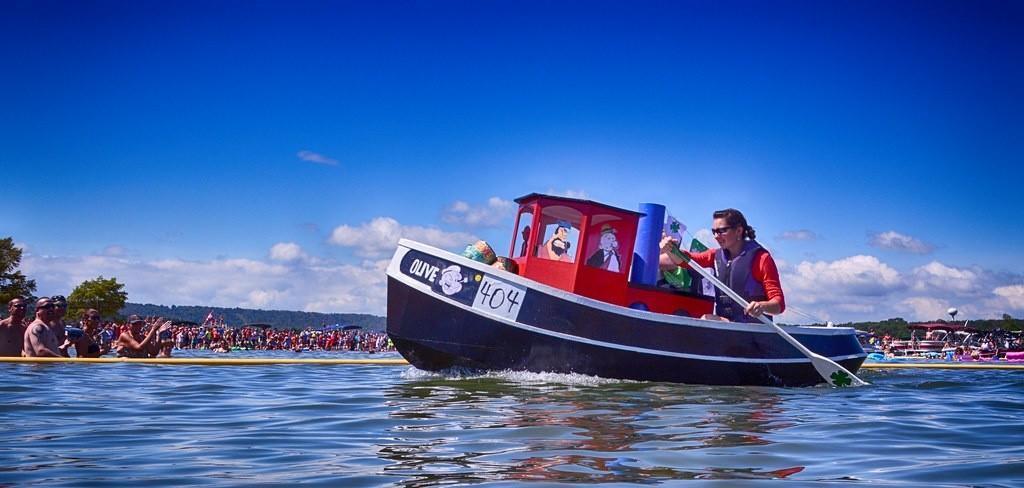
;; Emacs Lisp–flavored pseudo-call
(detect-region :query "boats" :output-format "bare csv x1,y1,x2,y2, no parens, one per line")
855,318,1024,363
385,192,869,387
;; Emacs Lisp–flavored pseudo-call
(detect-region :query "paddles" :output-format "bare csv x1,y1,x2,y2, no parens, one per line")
670,243,866,388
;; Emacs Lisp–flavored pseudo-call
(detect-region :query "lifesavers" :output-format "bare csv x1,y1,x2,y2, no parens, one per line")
1006,352,1024,362
954,354,972,361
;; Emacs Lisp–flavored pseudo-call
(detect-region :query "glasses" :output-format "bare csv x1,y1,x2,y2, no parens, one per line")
14,304,27,308
87,316,100,321
36,304,55,310
54,303,67,308
712,227,734,234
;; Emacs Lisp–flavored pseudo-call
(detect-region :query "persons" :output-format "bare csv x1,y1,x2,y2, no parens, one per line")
885,333,892,341
911,330,920,350
0,296,396,359
869,329,875,345
884,346,895,357
926,328,933,340
659,208,785,323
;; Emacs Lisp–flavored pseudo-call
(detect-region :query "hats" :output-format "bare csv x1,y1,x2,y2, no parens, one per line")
312,333,316,338
129,314,143,324
52,295,65,301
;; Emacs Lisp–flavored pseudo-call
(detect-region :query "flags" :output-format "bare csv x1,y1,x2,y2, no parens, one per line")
203,312,215,325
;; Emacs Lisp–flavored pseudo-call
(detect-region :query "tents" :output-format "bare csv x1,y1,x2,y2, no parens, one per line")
322,324,361,330
906,323,977,343
